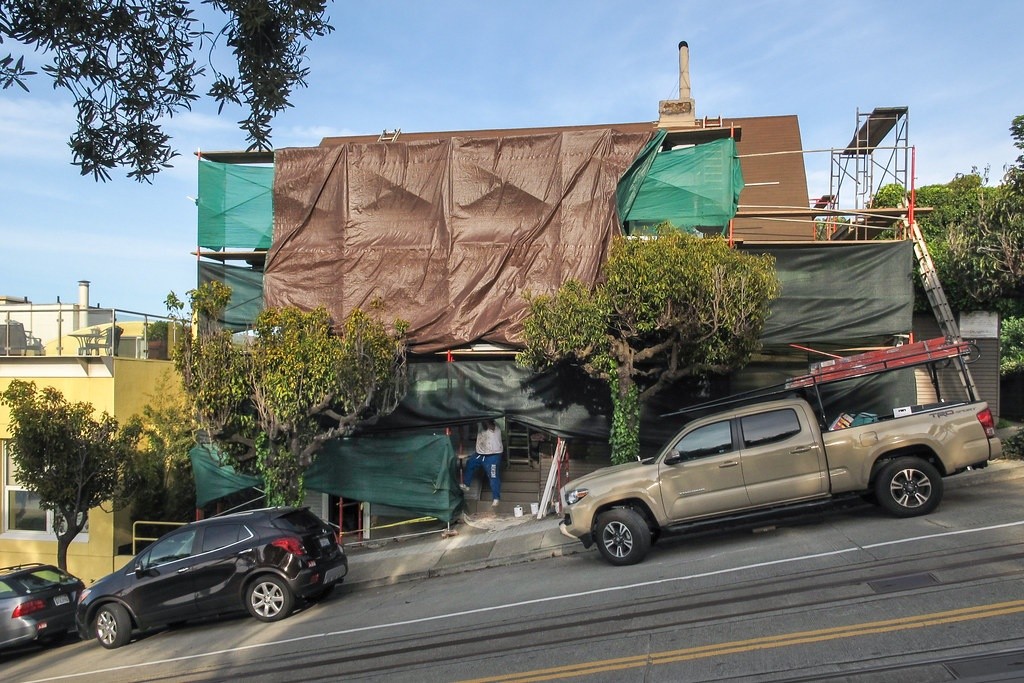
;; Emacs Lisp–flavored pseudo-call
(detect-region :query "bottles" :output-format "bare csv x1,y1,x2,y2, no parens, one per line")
514,504,523,517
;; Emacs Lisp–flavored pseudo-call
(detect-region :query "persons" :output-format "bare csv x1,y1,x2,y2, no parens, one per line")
459,418,504,507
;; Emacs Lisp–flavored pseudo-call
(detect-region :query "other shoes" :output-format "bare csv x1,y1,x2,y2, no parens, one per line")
459,484,470,491
491,499,499,507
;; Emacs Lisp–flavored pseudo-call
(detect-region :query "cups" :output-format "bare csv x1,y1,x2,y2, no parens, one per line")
531,503,539,515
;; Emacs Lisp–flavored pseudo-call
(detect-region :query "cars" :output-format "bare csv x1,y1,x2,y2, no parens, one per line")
0,562,85,651
73,505,349,650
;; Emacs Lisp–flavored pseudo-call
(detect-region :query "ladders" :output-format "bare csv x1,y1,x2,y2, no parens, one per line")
905,218,983,402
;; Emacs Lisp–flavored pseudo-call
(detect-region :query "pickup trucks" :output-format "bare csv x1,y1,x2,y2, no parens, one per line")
559,395,1003,567
